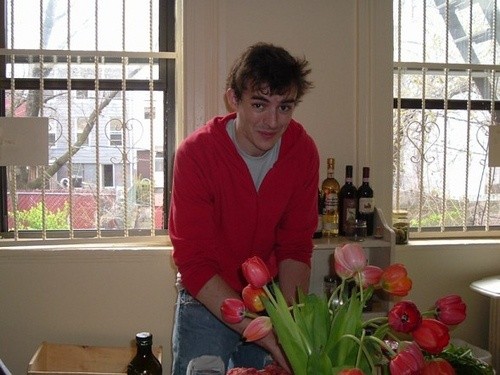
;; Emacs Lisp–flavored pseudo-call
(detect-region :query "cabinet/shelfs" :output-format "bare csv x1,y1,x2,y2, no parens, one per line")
311,208,396,312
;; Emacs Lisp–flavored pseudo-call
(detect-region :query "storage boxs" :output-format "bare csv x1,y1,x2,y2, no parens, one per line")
27,341,162,375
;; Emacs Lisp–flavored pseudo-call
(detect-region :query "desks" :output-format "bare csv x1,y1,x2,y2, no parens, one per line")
469,275,500,375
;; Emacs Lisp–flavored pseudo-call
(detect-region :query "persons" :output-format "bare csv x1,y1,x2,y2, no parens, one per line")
169,42,322,375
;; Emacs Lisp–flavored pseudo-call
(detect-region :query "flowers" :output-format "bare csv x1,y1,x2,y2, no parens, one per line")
220,236,466,375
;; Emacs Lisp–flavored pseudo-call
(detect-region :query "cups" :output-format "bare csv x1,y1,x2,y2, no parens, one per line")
345,220,367,244
392,210,408,244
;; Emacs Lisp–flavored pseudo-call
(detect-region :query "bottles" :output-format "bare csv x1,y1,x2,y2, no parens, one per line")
356,167,374,236
312,188,322,239
127,332,162,375
339,165,358,237
322,158,340,238
